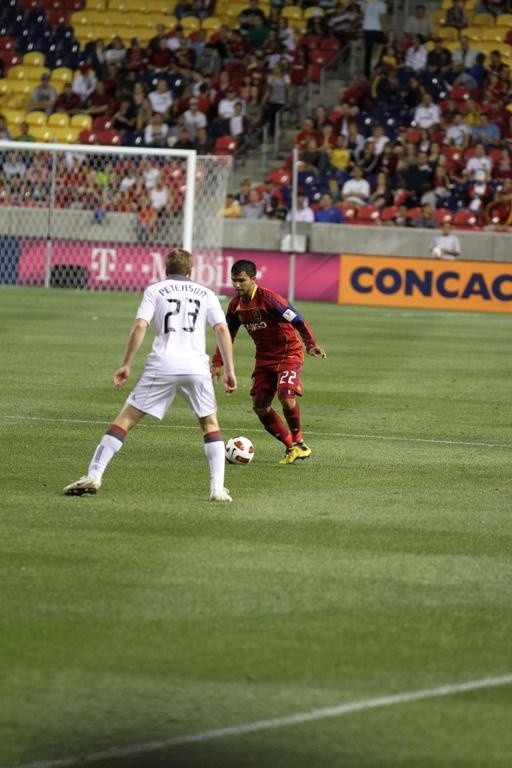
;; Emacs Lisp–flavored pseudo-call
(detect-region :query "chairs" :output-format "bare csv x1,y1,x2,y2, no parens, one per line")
0,0,323,146
425,1,511,76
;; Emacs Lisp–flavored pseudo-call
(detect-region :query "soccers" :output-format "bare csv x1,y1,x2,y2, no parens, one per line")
225,435,254,464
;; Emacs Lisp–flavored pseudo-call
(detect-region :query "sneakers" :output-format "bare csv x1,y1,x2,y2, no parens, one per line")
210,488,233,502
292,439,312,460
278,447,299,465
63,476,100,496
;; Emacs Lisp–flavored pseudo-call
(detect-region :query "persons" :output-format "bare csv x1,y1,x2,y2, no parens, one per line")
63,249,237,501
428,223,461,261
1,1,511,234
209,260,327,465
